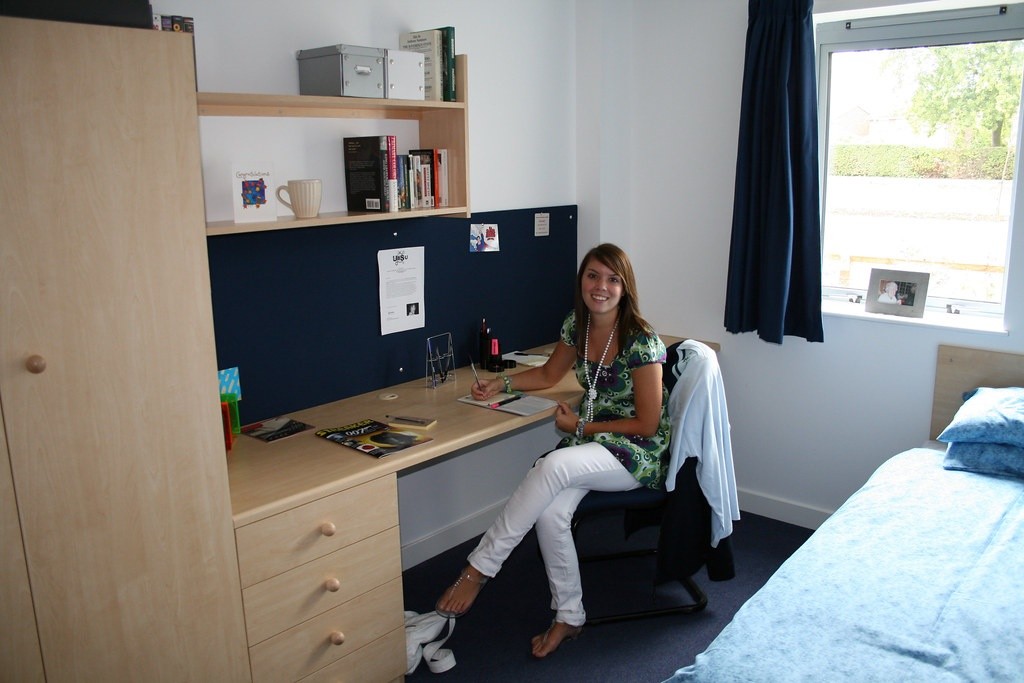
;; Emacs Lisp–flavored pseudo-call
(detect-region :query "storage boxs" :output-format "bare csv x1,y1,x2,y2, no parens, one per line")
383,49,425,99
296,44,385,97
162,15,198,92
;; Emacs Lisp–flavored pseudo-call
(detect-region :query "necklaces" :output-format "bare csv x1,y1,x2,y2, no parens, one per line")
585,310,621,422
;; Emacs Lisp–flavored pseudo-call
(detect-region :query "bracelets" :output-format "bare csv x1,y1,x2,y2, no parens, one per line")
576,419,587,437
499,373,511,393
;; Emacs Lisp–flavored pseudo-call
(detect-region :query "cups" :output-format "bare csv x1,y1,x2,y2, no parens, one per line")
275,179,322,217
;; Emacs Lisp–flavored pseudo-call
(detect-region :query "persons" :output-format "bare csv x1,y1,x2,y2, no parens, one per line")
438,244,669,656
409,305,416,315
502,351,550,367
476,234,488,251
878,282,899,304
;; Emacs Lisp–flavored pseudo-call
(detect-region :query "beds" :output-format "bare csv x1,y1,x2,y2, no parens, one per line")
665,345,1024,683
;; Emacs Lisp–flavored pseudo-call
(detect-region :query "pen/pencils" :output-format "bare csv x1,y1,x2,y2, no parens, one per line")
487,395,515,406
385,414,425,424
490,395,521,409
514,353,543,356
466,351,482,392
482,318,491,354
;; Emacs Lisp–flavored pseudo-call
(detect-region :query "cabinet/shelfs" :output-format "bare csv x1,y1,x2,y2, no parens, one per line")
0,13,470,682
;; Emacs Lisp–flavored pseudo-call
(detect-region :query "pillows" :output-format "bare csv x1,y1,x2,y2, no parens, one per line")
935,386,1024,478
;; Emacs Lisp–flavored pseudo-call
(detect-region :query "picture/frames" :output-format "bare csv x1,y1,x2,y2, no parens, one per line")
864,269,929,318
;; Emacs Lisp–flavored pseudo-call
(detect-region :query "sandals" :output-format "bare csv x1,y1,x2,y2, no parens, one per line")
435,565,490,618
531,620,583,659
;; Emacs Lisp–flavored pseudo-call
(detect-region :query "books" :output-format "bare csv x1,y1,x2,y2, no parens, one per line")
388,416,437,430
240,415,316,443
400,27,456,102
343,136,449,213
315,419,434,459
456,390,558,416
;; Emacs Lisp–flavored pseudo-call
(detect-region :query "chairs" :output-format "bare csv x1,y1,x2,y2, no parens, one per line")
579,342,724,623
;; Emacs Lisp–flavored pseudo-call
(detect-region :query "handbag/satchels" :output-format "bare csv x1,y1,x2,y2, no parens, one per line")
404,610,456,675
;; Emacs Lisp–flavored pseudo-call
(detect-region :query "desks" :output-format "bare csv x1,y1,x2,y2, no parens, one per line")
303,331,720,478
